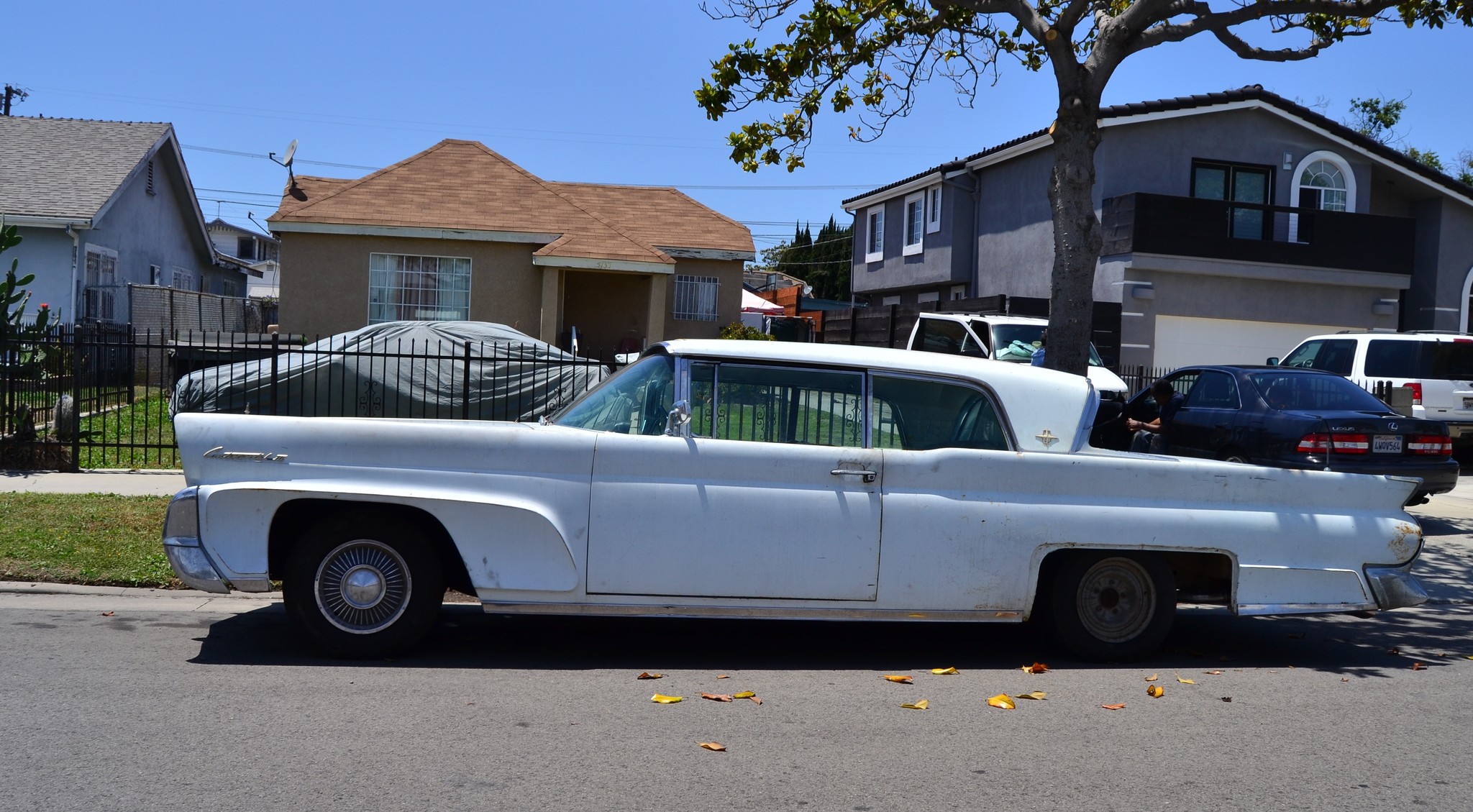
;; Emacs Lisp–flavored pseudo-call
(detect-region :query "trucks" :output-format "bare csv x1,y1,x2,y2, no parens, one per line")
740,311,816,343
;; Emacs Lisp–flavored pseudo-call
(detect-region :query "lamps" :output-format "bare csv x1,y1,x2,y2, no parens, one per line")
1372,303,1394,314
1133,289,1156,299
1283,151,1293,171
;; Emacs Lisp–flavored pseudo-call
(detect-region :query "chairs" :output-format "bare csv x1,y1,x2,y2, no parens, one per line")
1268,385,1297,409
1206,380,1230,407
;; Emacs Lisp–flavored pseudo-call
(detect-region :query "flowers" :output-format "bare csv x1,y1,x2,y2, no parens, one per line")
263,294,279,309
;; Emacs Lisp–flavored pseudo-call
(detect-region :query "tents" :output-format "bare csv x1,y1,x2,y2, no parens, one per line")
742,289,784,316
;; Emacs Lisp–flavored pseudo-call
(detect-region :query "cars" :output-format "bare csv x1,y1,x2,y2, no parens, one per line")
172,336,1429,657
1097,359,1460,510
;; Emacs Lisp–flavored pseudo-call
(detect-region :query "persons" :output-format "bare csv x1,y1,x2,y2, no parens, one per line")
1124,378,1185,456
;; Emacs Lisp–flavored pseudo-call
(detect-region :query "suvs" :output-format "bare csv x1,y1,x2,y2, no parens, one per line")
1264,329,1473,439
905,312,1129,423
